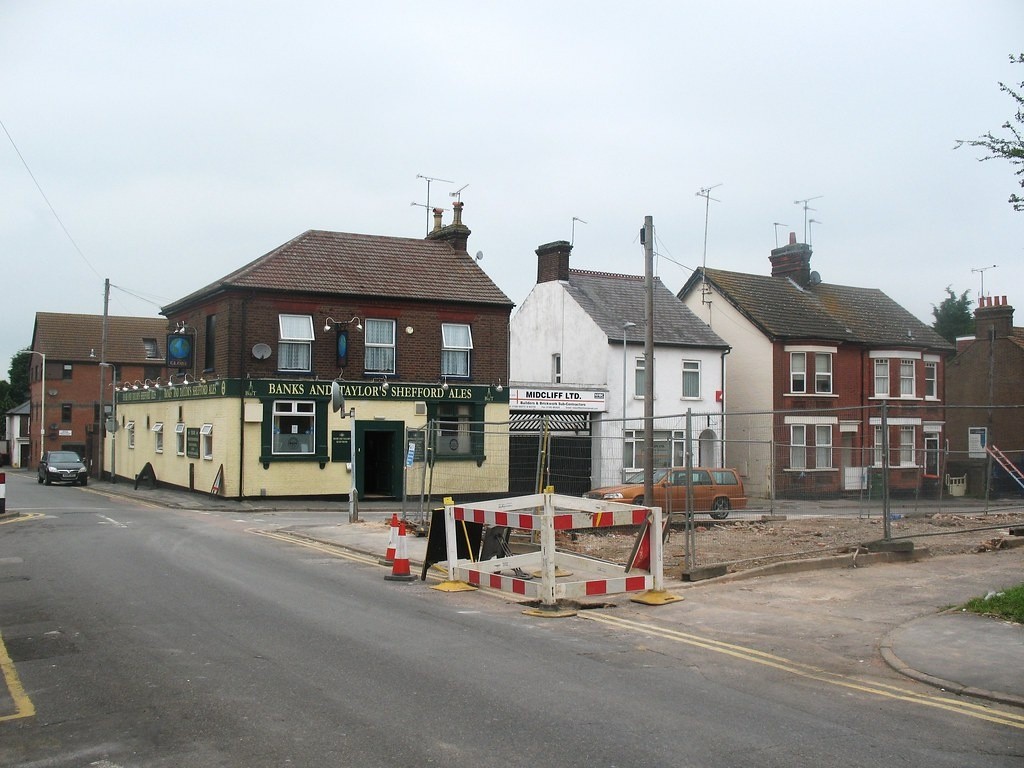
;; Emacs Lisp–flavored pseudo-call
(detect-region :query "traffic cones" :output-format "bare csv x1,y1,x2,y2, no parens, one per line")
379,513,399,567
384,522,418,582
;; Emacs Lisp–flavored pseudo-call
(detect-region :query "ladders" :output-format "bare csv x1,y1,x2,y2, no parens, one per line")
985,445,1024,489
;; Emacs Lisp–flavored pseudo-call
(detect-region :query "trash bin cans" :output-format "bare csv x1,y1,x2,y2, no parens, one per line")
870,473,885,497
922,474,940,500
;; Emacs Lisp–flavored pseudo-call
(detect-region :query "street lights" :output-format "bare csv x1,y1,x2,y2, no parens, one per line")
99,363,116,482
622,322,637,471
18,351,45,460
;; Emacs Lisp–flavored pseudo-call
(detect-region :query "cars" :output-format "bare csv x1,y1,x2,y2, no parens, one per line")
583,467,748,519
37,451,88,487
